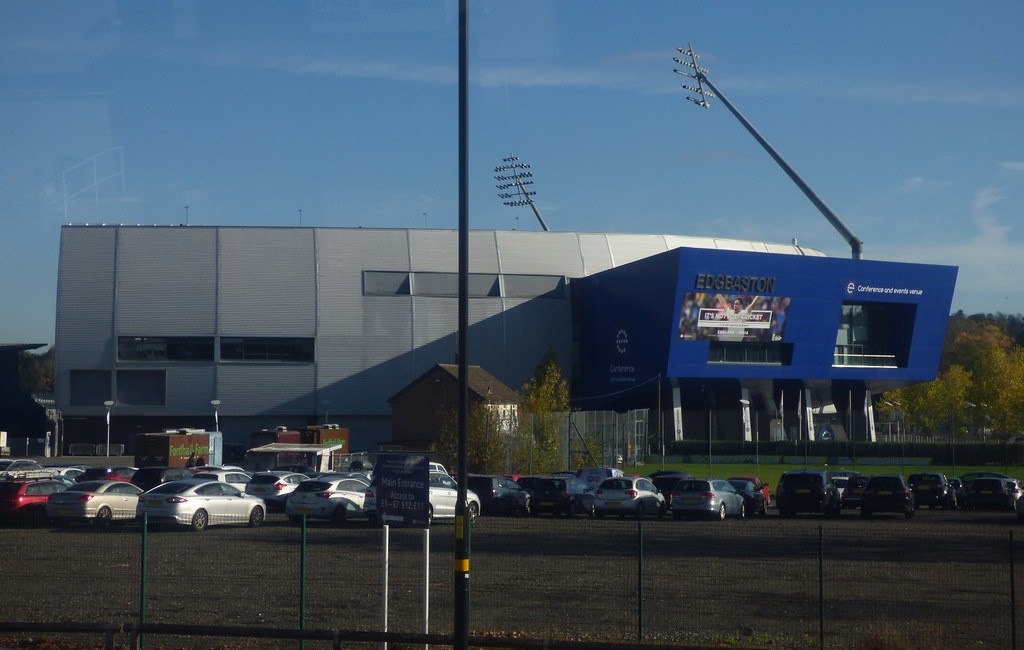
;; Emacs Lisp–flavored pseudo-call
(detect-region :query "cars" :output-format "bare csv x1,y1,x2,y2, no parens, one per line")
949,473,1024,516
0,459,481,525
2,480,66,526
451,470,770,520
776,470,867,516
48,481,141,530
135,481,267,530
910,473,948,508
865,475,913,519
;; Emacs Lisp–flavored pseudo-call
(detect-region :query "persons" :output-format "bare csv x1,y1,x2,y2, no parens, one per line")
678,292,736,340
743,295,790,342
188,452,196,468
195,455,206,470
717,293,761,343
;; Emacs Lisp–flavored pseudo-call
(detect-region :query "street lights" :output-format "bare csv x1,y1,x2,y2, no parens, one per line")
673,42,862,259
493,152,550,231
211,400,222,431
104,400,114,459
